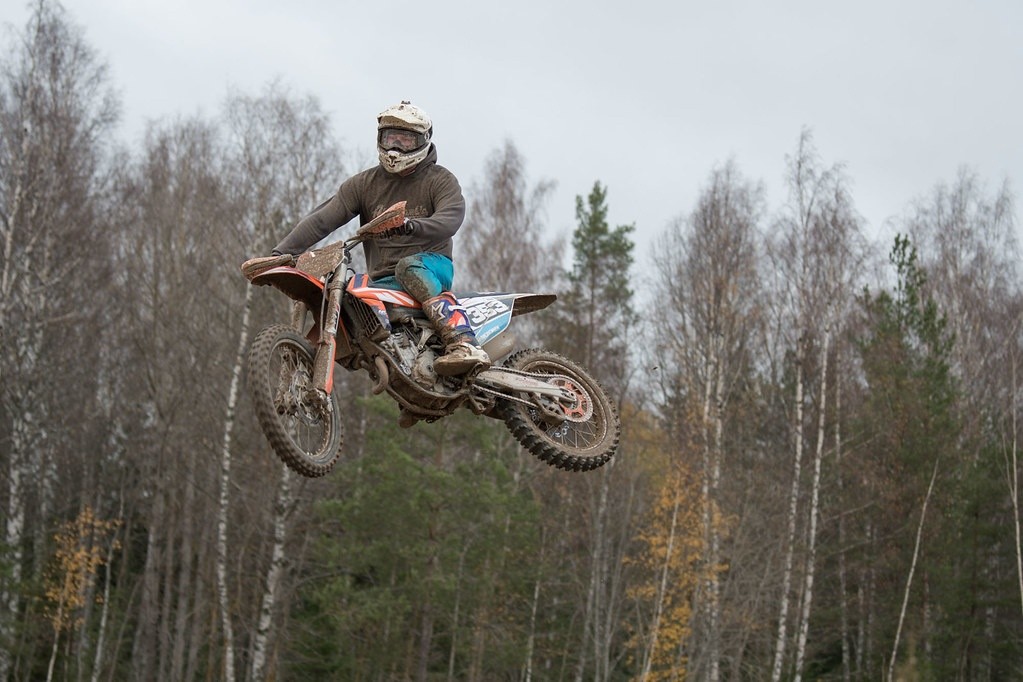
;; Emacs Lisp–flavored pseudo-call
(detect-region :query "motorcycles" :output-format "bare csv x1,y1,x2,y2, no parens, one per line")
241,202,620,479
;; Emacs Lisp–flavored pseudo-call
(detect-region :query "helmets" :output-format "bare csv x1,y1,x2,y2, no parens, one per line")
377,103,434,174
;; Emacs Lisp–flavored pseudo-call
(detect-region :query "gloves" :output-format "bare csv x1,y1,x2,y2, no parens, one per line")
380,217,414,239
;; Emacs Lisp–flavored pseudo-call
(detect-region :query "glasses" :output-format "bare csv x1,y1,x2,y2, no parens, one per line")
380,129,424,151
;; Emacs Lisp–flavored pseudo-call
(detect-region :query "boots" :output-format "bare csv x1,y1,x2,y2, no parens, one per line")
422,292,491,376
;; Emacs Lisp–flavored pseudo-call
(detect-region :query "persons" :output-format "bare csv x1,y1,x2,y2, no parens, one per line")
270,101,490,427
